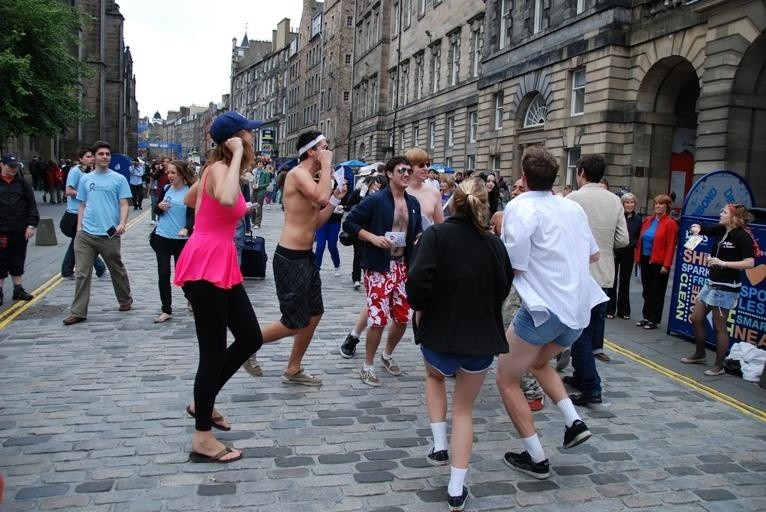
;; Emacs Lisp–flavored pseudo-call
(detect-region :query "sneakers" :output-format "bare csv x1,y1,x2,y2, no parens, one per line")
680,357,707,364
282,369,321,386
426,447,449,466
13,287,33,300
340,333,401,386
504,450,550,479
243,352,262,376
596,353,609,361
563,420,591,448
448,484,469,511
556,347,571,370
119,296,133,311
63,316,84,324
636,320,657,329
704,368,725,375
155,313,173,322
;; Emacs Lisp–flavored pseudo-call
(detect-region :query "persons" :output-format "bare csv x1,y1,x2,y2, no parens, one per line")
174,111,346,464
340,146,642,512
633,194,680,330
1,141,198,326
681,204,756,377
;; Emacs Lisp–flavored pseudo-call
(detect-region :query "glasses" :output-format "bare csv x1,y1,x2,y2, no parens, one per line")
399,168,413,175
418,163,430,168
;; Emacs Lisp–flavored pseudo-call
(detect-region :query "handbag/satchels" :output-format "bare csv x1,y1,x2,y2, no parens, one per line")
60,212,77,238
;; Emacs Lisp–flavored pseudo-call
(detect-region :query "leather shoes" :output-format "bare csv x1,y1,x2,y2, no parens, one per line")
563,373,602,404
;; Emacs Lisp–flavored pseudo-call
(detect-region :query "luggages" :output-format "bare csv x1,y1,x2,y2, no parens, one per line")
242,229,267,277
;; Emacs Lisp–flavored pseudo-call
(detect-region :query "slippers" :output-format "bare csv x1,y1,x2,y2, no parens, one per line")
190,443,242,463
187,402,231,431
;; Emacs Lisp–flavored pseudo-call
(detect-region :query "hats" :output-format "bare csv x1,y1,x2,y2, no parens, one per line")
1,155,19,167
210,112,262,143
